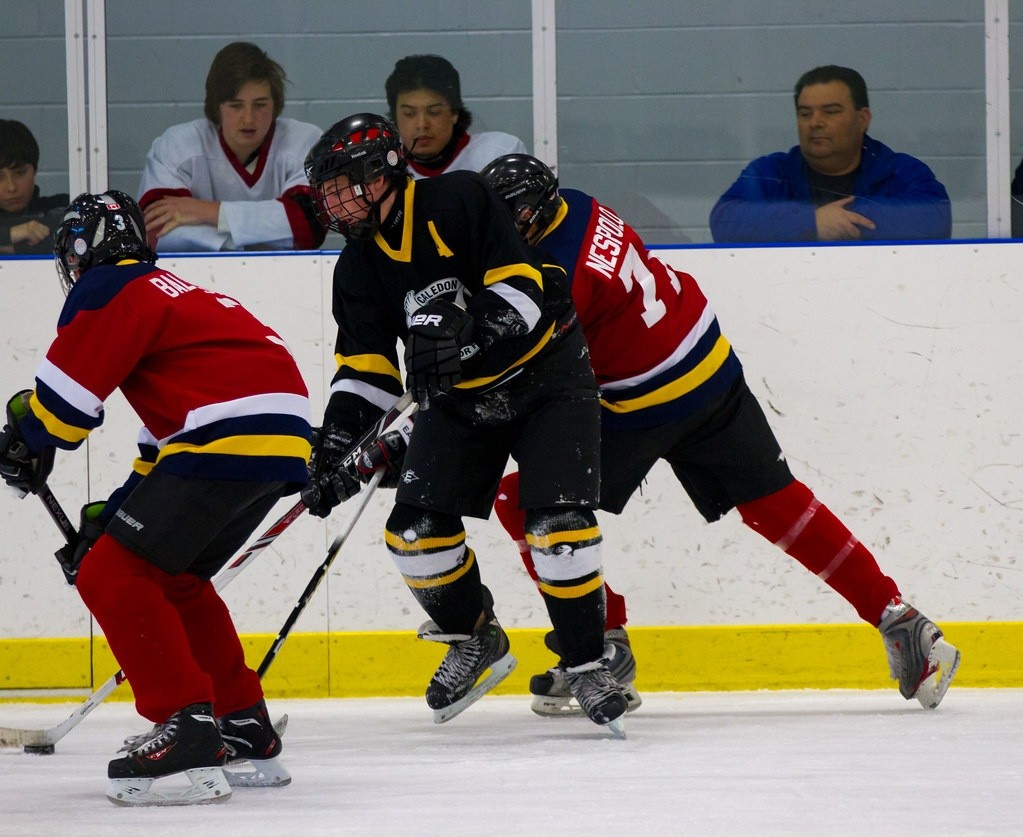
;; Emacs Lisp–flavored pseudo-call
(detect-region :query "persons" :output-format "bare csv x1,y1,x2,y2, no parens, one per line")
0,189,311,779
386,55,527,180
303,112,628,725
480,154,943,700
0,119,71,254
709,64,951,243
137,41,327,252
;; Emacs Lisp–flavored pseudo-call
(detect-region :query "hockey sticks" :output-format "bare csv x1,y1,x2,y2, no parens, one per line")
254,467,384,739
9,441,81,544
0,387,413,749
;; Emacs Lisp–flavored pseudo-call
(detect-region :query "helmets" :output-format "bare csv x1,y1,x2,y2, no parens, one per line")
54,190,159,286
480,154,563,228
303,113,401,186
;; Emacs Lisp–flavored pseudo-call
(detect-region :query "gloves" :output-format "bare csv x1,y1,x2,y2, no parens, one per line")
404,299,473,411
0,389,56,496
300,426,362,519
54,501,107,585
349,413,414,489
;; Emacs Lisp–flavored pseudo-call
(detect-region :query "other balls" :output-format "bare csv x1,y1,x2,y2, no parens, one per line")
24,744,56,755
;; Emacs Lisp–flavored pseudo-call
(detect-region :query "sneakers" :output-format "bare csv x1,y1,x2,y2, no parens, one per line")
217,699,292,786
106,701,232,809
417,584,518,724
878,596,961,711
529,625,642,717
544,630,629,739
